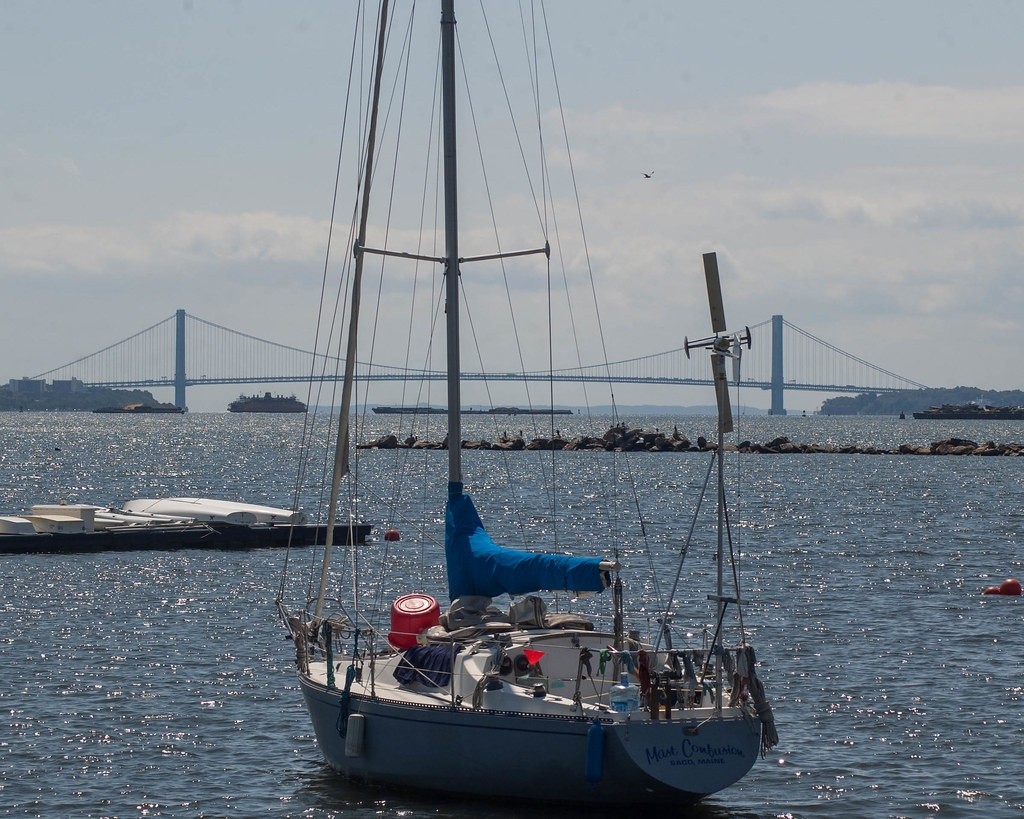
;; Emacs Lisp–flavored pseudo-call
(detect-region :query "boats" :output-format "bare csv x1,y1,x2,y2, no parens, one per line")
225,389,309,413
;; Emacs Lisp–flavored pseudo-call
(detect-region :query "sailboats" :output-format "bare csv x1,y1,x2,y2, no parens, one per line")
275,0,780,807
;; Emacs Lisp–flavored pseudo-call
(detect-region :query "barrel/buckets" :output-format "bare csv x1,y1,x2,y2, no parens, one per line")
387,594,440,651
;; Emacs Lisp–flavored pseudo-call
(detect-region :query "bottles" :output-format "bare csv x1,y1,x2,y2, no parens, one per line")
610,672,641,717
